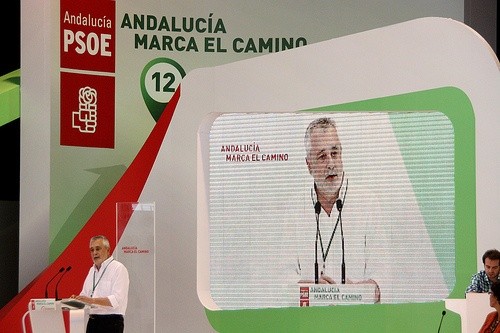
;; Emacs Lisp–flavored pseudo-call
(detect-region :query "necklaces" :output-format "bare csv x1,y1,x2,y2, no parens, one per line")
310,178,348,270
90,259,115,297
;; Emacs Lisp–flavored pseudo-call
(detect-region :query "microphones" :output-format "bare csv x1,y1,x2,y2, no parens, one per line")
45,267,64,298
336,199,345,284
56,266,72,301
438,311,446,333
315,201,321,285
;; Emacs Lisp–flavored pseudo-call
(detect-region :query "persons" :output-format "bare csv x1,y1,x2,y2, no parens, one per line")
70,235,129,333
464,249,500,299
477,279,500,333
279,117,393,304
